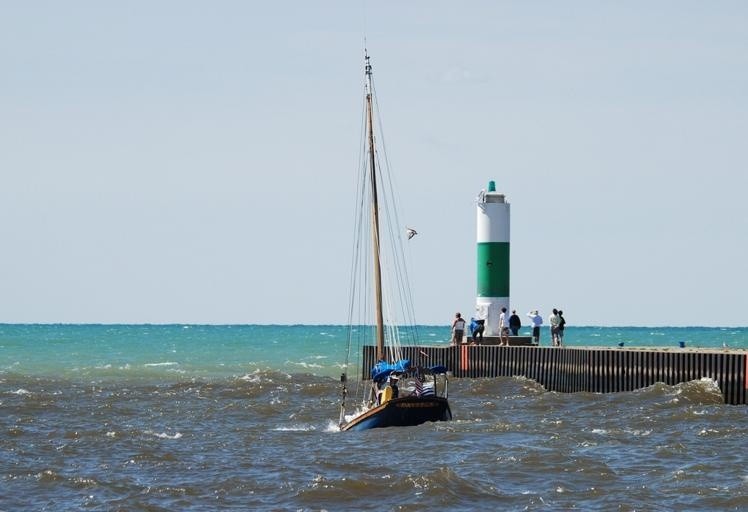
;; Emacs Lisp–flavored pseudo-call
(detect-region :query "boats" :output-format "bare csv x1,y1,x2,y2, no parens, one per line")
339,33,452,433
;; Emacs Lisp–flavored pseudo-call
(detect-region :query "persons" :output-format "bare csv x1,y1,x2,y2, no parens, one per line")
451,313,466,345
468,317,485,344
497,308,511,346
509,310,522,336
557,311,567,345
526,309,544,346
550,309,563,347
